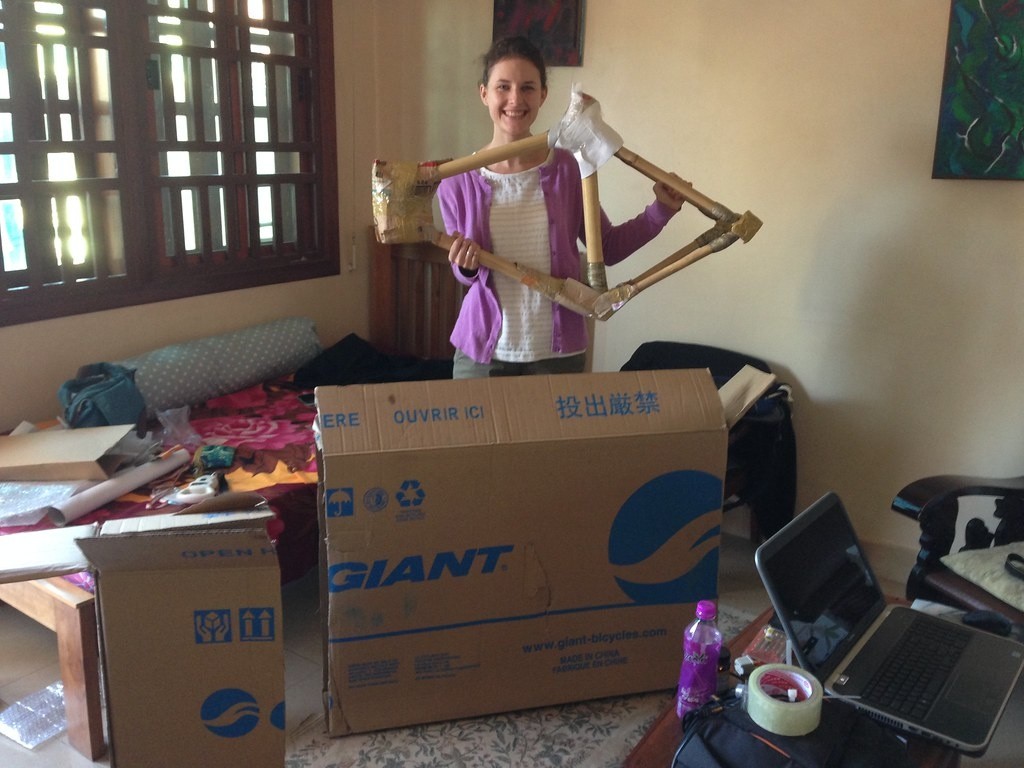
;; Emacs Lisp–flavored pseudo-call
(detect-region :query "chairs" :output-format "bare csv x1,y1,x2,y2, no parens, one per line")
891,475,1024,639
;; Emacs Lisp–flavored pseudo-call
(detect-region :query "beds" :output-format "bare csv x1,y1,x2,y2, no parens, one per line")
0,224,472,763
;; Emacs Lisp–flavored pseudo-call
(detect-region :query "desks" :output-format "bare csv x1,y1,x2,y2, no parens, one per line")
624,599,916,768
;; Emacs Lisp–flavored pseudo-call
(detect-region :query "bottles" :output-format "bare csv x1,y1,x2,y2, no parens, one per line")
676,601,721,719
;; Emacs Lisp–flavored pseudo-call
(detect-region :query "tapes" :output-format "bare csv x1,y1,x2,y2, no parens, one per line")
746,663,823,736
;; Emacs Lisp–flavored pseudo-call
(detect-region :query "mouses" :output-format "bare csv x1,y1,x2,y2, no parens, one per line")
961,610,1012,637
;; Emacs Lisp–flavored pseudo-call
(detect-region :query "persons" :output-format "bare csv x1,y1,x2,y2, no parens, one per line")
437,36,692,379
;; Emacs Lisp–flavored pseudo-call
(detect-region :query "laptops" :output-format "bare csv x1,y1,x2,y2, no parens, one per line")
755,491,1024,757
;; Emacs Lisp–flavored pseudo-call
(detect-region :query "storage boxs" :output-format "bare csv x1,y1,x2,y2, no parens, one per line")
76,490,286,768
312,368,729,733
